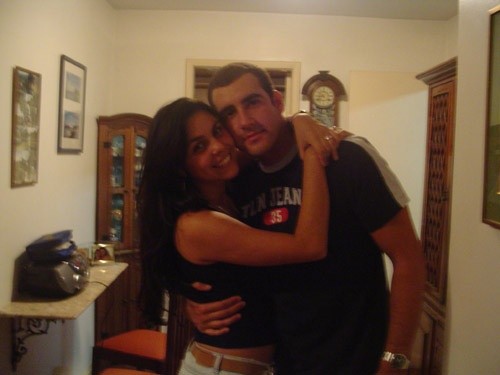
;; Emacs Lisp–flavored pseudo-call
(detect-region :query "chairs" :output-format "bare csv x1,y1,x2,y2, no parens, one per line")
91,292,190,375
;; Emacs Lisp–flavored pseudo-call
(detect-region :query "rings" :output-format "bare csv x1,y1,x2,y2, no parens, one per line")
325,135,333,141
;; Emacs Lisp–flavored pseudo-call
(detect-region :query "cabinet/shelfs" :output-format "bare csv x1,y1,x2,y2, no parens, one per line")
406,56,457,375
95,112,165,346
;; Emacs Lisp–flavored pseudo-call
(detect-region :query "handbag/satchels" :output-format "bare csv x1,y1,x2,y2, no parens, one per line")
24,229,89,301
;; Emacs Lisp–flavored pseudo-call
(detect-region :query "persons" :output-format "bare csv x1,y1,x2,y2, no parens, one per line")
99,247,113,260
138,97,354,375
184,61,427,375
94,249,102,260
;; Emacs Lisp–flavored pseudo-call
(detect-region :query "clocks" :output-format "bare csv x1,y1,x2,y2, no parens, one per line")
301,70,345,128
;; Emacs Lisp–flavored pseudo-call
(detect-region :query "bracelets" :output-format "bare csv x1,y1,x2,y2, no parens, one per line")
290,110,314,125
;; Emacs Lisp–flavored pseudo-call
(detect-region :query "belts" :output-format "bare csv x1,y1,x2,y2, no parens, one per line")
189,342,269,375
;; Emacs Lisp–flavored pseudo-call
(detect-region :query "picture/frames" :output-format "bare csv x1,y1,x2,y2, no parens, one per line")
56,55,86,154
482,4,500,232
11,65,41,189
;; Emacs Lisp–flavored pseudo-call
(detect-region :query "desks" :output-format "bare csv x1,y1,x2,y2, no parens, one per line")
0,259,129,375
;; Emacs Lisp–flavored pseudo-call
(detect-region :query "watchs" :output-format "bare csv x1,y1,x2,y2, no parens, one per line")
382,351,412,370
